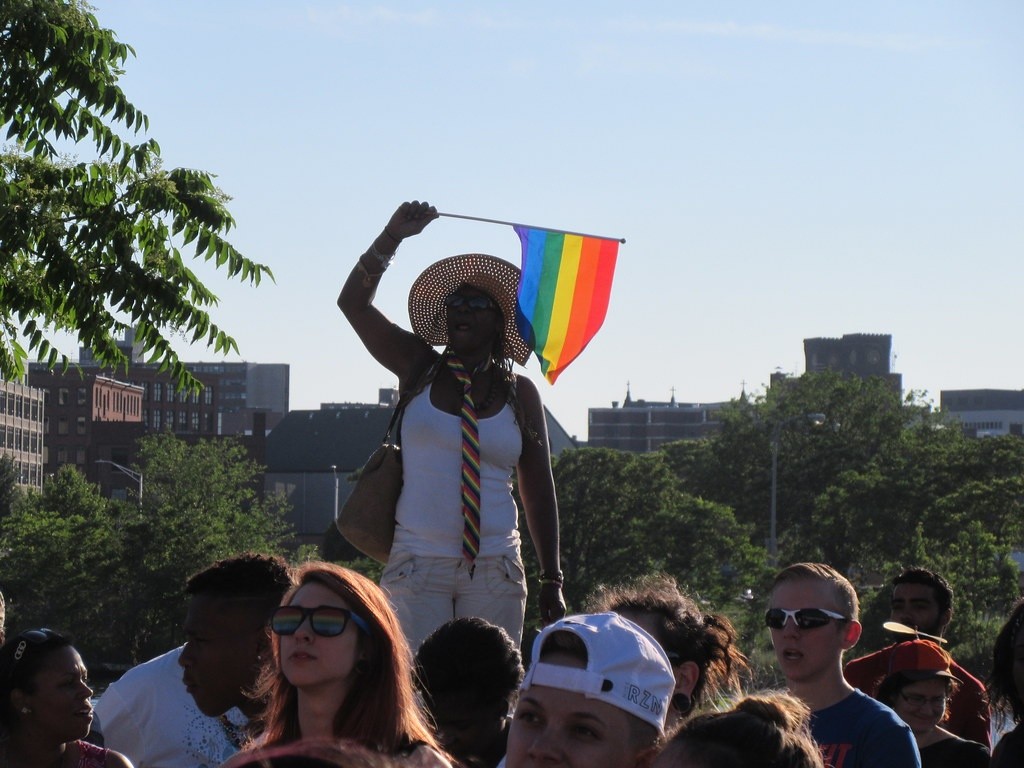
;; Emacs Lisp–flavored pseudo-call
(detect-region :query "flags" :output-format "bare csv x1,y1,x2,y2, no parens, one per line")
511,223,620,385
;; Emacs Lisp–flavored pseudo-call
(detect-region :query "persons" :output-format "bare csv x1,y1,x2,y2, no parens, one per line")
339,200,567,653
0,551,1024,768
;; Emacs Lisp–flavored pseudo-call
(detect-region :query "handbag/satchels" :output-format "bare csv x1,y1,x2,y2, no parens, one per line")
335,342,446,561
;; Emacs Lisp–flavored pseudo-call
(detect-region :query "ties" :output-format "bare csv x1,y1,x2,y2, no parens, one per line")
445,351,481,561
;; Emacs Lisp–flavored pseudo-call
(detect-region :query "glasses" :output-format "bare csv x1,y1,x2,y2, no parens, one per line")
764,606,848,631
897,689,950,707
270,603,363,638
9,626,58,686
445,293,498,314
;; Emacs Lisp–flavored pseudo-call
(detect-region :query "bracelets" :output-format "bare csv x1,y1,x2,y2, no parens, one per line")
538,575,564,583
539,569,563,575
370,241,397,269
540,580,563,587
385,227,403,245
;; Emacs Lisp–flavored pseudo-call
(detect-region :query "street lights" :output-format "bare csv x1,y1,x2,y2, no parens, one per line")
770,412,827,550
331,464,339,520
95,459,144,519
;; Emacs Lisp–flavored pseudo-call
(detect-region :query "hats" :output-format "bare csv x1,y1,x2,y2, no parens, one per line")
521,611,673,736
407,253,535,368
887,639,965,688
